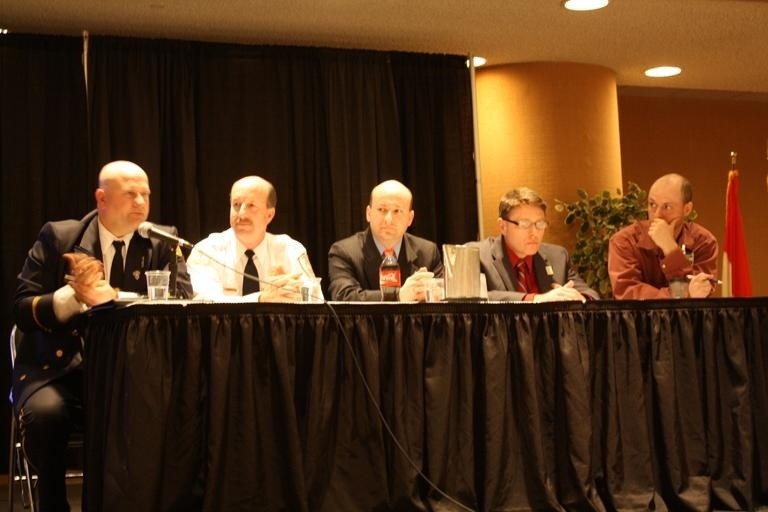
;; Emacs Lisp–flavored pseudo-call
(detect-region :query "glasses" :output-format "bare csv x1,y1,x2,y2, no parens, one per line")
506,218,549,229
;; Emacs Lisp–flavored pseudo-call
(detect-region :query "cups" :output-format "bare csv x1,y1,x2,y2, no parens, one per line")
423,277,444,302
300,277,321,302
145,271,170,302
669,281,689,298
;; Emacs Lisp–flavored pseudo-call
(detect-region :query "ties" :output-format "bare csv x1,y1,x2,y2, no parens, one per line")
110,241,124,290
516,259,528,294
242,250,260,296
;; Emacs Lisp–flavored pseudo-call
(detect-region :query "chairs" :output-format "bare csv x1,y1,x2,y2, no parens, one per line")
9,323,84,512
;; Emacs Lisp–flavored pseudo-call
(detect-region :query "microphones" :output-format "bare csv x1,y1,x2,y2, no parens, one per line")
138,221,192,255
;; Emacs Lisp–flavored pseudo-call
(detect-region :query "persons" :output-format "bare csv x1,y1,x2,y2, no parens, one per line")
185,176,325,305
608,173,719,299
12,161,194,512
471,187,600,303
327,180,445,302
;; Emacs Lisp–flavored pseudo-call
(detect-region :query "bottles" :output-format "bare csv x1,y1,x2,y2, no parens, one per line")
378,247,401,302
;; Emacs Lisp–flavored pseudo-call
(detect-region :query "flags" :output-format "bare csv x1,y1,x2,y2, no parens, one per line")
722,170,753,298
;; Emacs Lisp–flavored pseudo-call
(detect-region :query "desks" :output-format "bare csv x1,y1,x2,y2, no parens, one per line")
75,298,767,512
